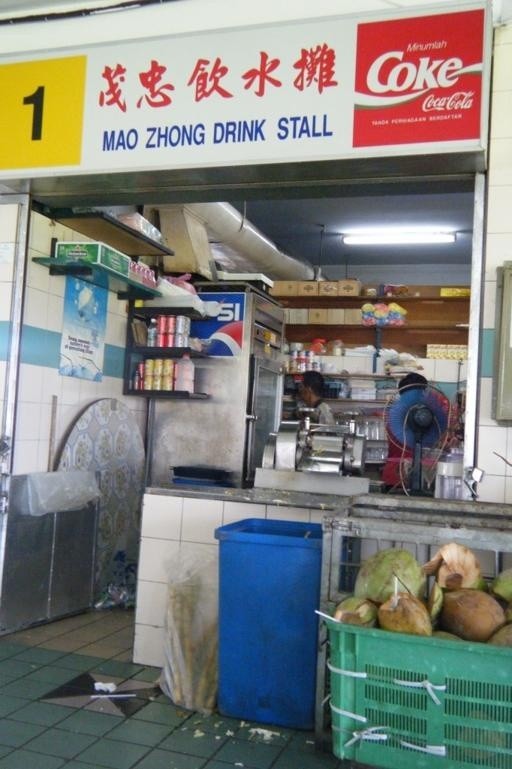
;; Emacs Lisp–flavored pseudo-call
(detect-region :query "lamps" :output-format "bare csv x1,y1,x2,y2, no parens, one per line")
342,234,456,245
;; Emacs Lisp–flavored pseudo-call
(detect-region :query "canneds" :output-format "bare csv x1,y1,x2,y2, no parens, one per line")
156,314,191,347
144,359,175,391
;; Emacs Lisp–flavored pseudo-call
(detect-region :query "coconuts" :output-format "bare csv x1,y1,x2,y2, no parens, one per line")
334,542,512,648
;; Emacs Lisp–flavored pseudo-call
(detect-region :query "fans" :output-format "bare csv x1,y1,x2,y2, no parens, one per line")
383,383,454,497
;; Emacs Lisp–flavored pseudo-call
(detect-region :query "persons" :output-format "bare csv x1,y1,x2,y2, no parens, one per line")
388,372,440,498
296,371,335,425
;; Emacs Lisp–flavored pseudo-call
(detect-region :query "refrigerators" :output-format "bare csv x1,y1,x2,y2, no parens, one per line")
144,280,286,487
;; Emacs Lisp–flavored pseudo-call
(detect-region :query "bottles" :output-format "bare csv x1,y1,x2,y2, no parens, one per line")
434,448,464,502
175,352,194,394
363,422,380,441
133,371,139,390
349,420,356,434
147,318,157,347
289,350,320,373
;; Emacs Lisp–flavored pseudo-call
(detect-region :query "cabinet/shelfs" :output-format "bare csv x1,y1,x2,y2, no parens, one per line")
123,266,207,408
272,286,470,463
32,212,174,300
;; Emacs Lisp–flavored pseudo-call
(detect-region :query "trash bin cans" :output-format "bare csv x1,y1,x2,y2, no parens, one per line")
213,519,347,730
169,465,237,488
